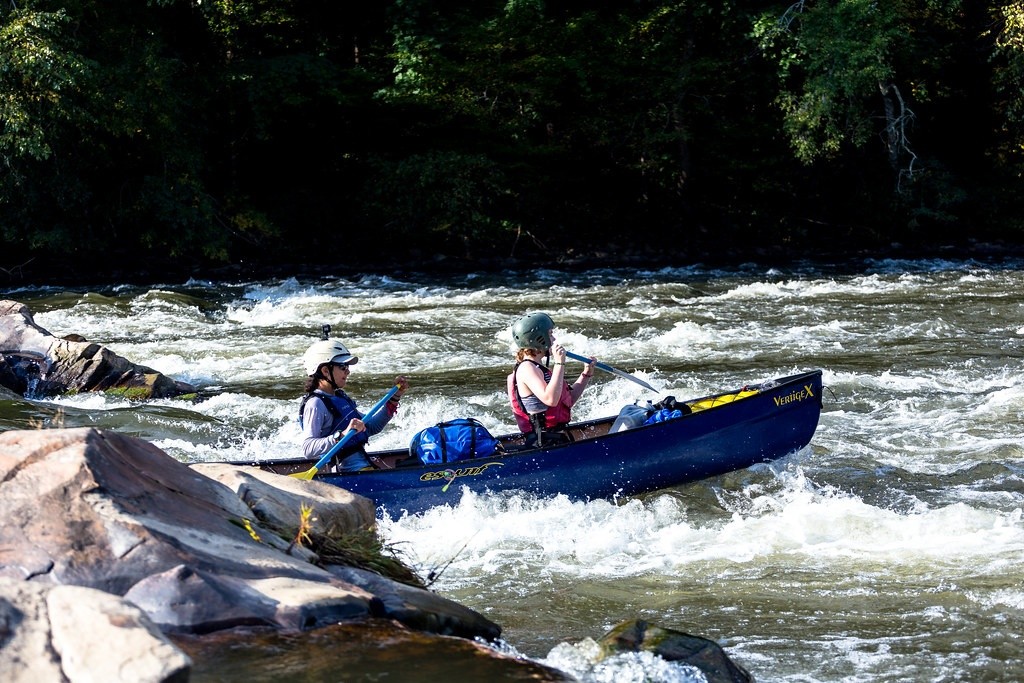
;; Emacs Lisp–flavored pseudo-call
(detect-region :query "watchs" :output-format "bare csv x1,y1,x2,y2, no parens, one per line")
333,430,342,441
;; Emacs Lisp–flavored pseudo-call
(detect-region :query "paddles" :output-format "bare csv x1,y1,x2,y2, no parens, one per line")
565,352,659,394
288,381,403,479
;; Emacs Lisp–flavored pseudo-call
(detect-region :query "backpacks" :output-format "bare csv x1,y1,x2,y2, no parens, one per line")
409,419,498,464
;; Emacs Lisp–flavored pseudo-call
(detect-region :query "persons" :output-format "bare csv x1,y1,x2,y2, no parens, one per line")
506,313,597,449
299,339,406,471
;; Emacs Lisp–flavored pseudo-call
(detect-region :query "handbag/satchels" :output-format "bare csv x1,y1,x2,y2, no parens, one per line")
608,399,650,434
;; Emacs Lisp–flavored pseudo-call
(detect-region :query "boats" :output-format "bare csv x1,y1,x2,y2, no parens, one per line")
227,369,825,526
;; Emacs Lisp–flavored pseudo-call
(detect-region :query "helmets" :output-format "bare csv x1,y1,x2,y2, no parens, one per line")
303,339,358,377
513,312,555,350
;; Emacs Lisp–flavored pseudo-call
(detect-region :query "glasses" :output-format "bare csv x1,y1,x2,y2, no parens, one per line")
333,363,349,370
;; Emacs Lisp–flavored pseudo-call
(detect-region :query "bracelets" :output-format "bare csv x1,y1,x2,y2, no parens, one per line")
554,362,566,366
